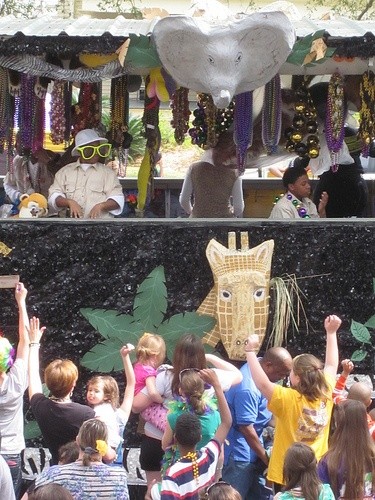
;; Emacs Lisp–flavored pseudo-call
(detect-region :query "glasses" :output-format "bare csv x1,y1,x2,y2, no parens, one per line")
177,367,201,383
76,143,112,160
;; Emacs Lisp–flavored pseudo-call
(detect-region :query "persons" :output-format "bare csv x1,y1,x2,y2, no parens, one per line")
86,373,124,448
311,152,370,219
2,127,67,217
1,281,31,500
106,312,375,500
178,148,244,218
0,455,18,500
34,104,108,177
24,316,97,467
47,129,124,220
19,420,130,500
268,167,329,220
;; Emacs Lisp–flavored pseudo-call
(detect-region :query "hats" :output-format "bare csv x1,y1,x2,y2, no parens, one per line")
70,128,108,159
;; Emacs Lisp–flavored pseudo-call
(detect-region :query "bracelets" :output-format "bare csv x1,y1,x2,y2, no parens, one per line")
28,342,40,347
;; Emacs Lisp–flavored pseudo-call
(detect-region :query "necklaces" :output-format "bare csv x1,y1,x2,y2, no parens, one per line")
49,396,70,402
25,158,42,194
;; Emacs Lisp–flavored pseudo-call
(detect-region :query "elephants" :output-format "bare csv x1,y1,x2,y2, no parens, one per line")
152,7,296,109
